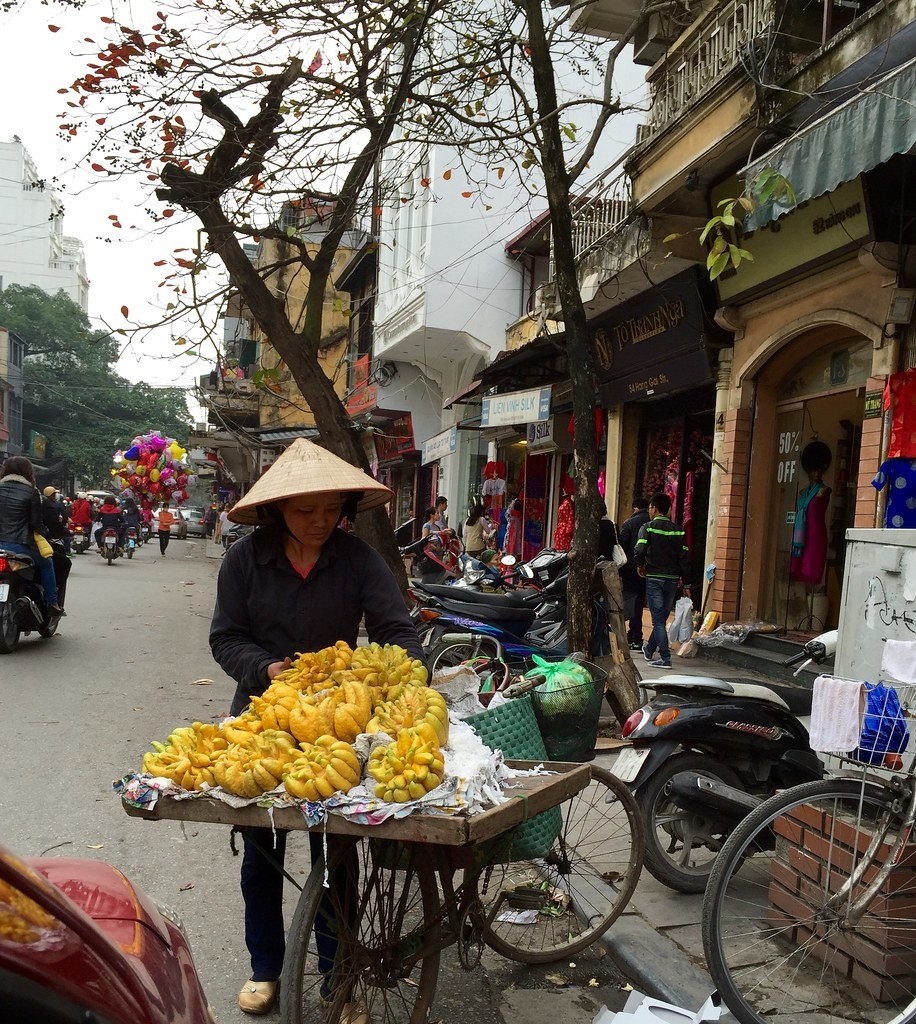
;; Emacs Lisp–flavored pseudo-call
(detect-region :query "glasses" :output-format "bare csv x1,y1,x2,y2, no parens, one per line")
646,507,652,511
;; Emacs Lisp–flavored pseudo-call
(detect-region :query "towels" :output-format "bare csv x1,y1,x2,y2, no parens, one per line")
809,676,869,752
881,639,916,686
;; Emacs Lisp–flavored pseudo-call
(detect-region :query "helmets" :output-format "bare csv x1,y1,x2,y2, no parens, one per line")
78,492,104,504
65,497,70,502
43,486,60,496
114,498,121,504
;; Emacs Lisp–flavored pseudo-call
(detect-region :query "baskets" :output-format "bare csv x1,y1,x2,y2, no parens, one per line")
816,675,916,775
506,655,607,761
363,692,564,869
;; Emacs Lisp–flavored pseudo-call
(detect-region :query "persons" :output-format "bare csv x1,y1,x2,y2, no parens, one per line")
788,469,832,585
422,496,453,560
597,502,620,561
208,438,432,1024
140,508,155,533
0,456,64,616
633,493,692,668
41,486,76,559
158,503,174,556
68,492,93,539
120,498,144,539
204,506,216,539
462,505,490,559
620,498,651,650
219,503,236,556
65,496,72,507
94,497,125,553
87,495,100,520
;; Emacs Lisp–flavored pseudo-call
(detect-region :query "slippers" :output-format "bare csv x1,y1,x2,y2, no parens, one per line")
238,975,277,1014
318,992,368,1024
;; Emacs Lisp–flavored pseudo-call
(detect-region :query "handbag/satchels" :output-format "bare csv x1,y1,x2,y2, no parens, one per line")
90,521,103,543
669,594,694,643
611,522,627,569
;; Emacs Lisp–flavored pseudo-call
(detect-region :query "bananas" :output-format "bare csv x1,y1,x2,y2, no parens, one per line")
141,641,449,803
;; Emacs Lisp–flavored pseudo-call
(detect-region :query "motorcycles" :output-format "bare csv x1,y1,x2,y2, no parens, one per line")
98,519,121,566
0,529,67,654
122,527,138,560
70,524,90,554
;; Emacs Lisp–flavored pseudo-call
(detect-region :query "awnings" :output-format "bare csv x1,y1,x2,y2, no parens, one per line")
736,57,916,233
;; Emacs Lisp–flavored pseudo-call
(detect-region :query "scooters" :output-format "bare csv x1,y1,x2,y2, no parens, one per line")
140,522,151,543
605,630,838,893
399,533,647,709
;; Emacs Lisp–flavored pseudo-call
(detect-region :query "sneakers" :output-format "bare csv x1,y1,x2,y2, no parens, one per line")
626,640,642,650
647,658,672,669
641,642,652,660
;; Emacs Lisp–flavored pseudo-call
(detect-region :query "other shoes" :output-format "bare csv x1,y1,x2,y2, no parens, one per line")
160,552,165,556
221,550,226,556
49,605,64,613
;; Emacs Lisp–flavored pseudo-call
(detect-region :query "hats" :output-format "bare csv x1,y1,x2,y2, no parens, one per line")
226,437,395,529
224,503,232,511
481,549,496,564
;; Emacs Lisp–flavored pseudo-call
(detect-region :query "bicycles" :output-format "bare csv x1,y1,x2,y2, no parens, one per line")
701,637,916,1024
280,632,647,1024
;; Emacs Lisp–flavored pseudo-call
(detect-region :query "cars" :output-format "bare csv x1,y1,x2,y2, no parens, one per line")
0,845,215,1024
178,506,207,538
151,507,190,540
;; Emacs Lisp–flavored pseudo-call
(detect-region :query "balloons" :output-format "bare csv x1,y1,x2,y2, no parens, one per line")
110,430,198,510
